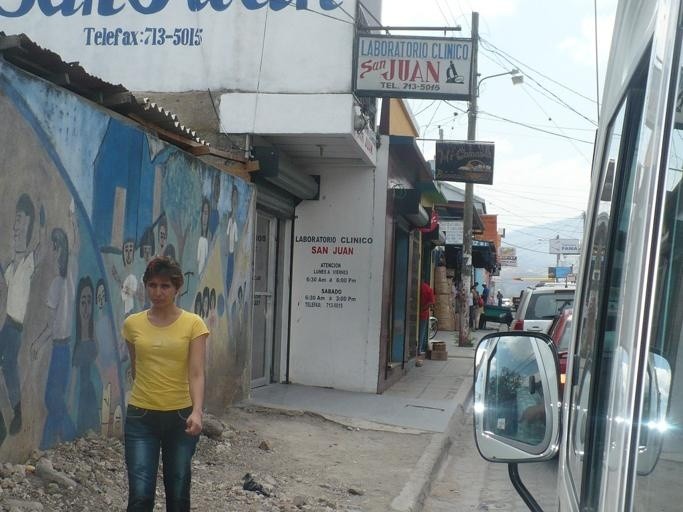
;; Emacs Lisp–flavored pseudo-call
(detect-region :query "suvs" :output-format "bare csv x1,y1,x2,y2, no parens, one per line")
510,282,576,395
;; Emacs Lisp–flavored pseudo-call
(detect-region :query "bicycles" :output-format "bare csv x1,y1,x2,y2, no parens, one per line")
428,317,439,339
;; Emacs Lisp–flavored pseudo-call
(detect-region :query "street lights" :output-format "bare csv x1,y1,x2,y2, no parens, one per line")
468,68,519,117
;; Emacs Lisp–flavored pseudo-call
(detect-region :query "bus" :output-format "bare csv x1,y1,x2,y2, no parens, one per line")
473,1,681,511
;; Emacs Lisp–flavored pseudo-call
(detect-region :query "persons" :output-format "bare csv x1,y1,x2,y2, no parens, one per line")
496,291,503,306
418,270,437,354
447,274,489,330
121,258,209,512
